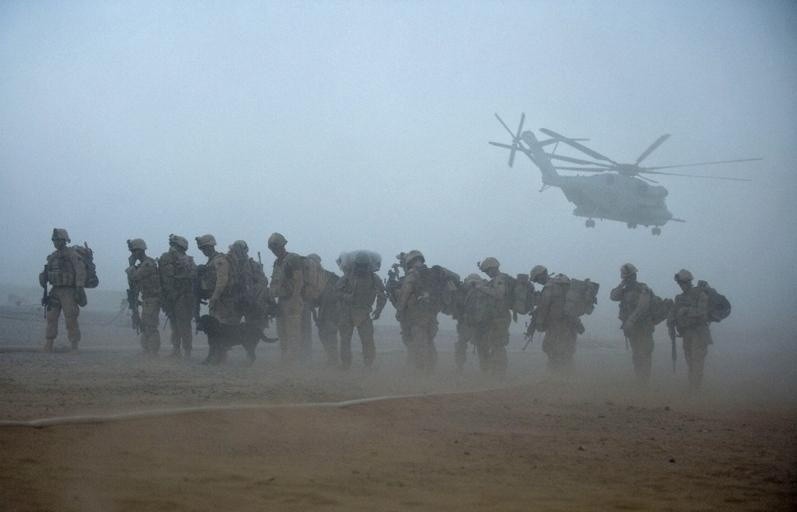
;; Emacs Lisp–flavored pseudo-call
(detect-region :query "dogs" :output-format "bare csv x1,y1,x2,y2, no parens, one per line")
195,314,279,369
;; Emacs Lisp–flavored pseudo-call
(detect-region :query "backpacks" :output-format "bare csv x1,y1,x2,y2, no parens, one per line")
283,252,327,301
334,250,382,274
548,277,599,316
499,271,535,315
74,245,99,289
427,265,461,315
698,280,732,322
145,257,177,306
647,291,667,325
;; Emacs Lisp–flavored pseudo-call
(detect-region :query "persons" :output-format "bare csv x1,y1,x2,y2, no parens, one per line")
668,268,713,386
38,227,86,351
609,262,656,374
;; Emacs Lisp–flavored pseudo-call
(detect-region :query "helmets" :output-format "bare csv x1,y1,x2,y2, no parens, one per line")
479,257,500,271
620,263,637,278
229,240,249,253
674,269,694,282
196,234,216,248
529,265,547,281
354,251,372,265
169,234,189,250
267,233,287,249
405,250,425,265
129,238,147,250
51,228,69,242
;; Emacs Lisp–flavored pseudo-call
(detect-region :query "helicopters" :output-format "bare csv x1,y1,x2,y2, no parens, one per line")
490,110,768,236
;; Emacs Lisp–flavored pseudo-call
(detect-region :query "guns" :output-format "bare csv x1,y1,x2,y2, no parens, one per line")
128,284,142,336
667,322,681,372
194,294,208,336
521,311,537,349
257,252,278,316
42,265,50,318
618,296,629,351
